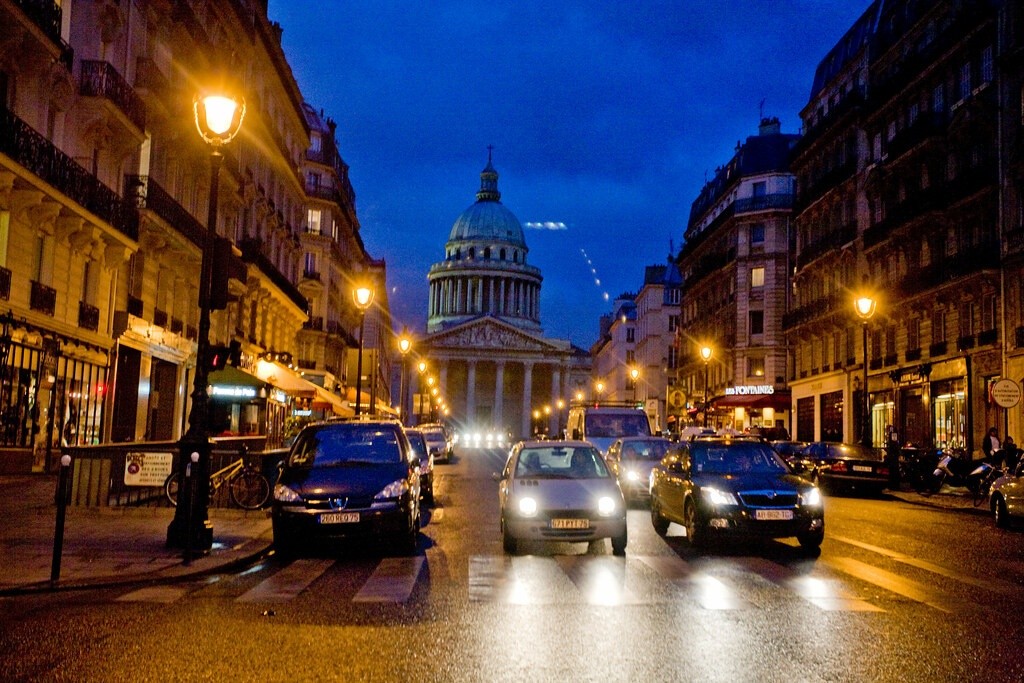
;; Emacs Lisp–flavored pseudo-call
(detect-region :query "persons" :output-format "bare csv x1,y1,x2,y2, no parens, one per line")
362,436,397,461
649,445,664,458
884,425,902,481
624,445,637,458
568,450,594,477
748,424,789,441
304,447,330,465
982,427,1024,475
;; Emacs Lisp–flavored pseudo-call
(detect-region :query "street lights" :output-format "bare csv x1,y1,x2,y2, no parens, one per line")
533,390,584,437
164,86,245,550
398,333,413,421
698,347,714,425
596,381,603,398
418,361,450,427
631,365,640,405
855,296,877,446
351,276,374,416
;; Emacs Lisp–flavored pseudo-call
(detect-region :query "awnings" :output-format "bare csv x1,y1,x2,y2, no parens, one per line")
666,393,792,423
208,362,274,390
272,367,386,417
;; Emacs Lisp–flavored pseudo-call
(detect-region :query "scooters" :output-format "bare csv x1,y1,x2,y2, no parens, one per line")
922,444,1001,496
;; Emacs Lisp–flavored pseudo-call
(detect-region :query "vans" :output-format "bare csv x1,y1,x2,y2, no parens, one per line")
564,407,651,463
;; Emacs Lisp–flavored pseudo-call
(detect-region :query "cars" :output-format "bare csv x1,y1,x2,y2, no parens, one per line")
791,443,888,495
271,417,424,541
606,436,671,502
415,422,454,463
492,439,627,552
988,452,1024,525
771,441,802,469
650,436,825,549
354,429,434,499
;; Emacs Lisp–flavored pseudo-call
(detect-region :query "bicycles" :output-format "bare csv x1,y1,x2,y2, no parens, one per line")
165,444,271,510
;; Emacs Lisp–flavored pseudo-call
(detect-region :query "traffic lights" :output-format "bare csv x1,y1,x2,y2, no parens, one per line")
206,343,229,368
212,235,245,310
